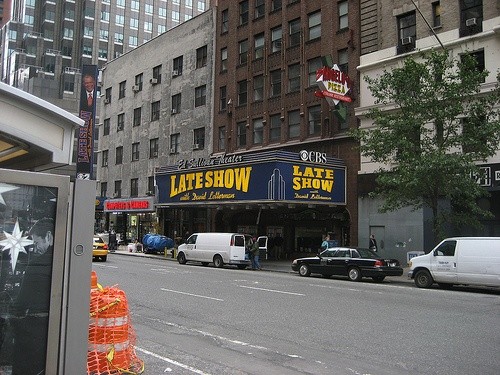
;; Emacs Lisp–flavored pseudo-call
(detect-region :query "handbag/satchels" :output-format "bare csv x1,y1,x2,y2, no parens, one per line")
371,246,377,252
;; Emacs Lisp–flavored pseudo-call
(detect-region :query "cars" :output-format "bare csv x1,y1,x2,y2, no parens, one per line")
92,235,109,262
290,246,403,283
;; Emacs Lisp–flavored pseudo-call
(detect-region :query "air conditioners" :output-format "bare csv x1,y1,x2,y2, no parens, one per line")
170,148,176,154
402,37,413,45
171,109,177,114
104,98,109,103
132,85,139,90
104,162,107,166
192,144,201,149
147,190,153,195
466,18,478,27
152,152,158,157
172,71,178,77
113,193,117,197
150,78,157,84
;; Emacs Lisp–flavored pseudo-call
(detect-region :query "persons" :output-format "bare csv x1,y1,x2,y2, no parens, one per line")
82,74,96,109
321,234,330,251
369,235,378,253
9,218,55,375
248,237,261,270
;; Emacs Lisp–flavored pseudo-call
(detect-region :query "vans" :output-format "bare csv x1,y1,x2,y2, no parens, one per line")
408,237,500,294
176,232,269,269
